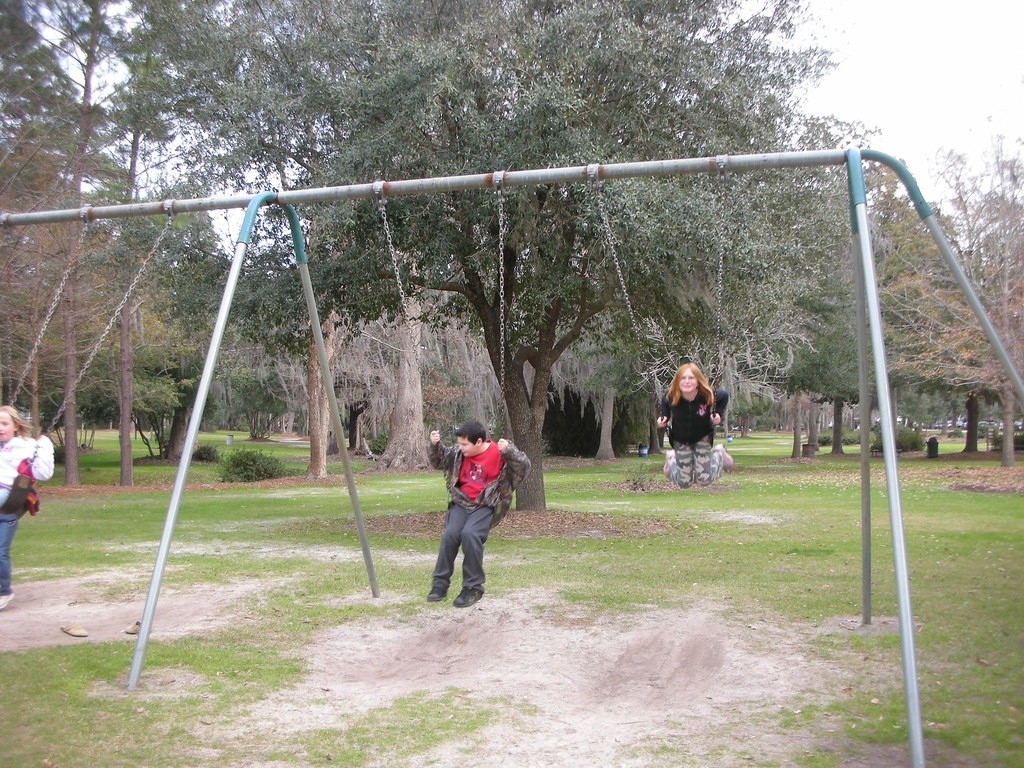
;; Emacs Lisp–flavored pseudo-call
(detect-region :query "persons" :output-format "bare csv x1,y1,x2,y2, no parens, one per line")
426,421,531,608
0,405,55,610
657,363,733,489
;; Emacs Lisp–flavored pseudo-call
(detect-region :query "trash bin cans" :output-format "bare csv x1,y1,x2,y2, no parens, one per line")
727,435,733,443
802,444,814,457
927,437,939,458
639,442,648,458
226,434,234,445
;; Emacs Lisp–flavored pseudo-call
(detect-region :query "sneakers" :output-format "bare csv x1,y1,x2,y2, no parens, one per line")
454,586,483,607
0,593,14,609
427,586,446,601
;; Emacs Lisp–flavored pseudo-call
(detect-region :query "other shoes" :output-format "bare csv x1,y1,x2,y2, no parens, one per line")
61,624,86,636
125,620,141,634
714,444,734,473
663,450,675,480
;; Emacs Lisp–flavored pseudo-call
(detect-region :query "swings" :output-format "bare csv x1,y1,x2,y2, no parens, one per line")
370,167,510,528
1,195,177,516
586,153,730,451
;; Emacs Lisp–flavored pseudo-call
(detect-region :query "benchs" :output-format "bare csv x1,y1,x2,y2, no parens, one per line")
624,444,639,455
871,449,902,457
